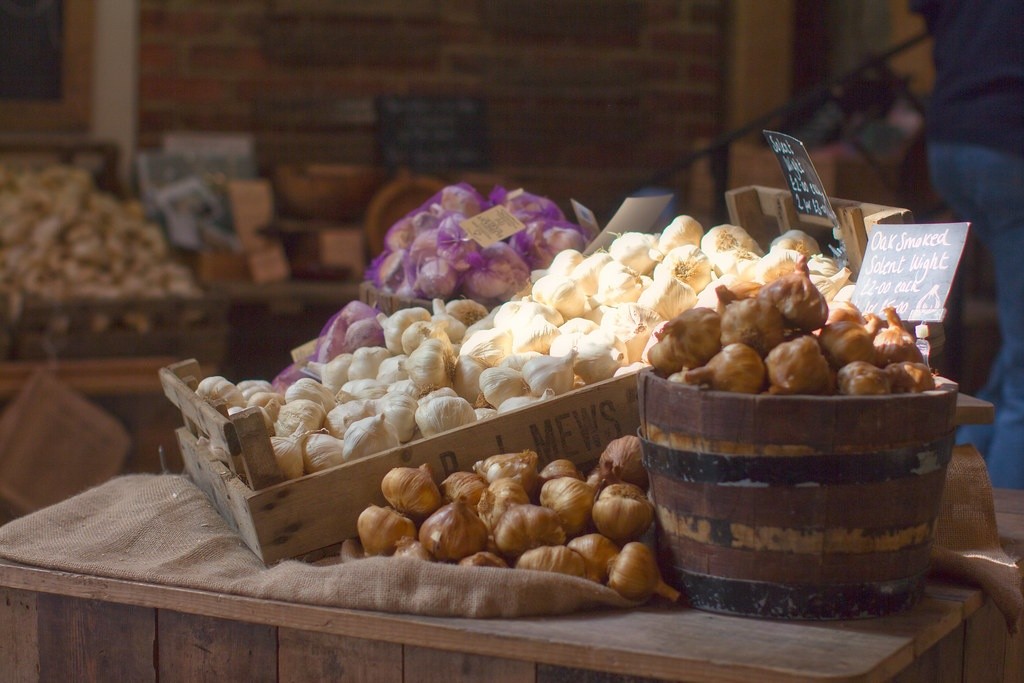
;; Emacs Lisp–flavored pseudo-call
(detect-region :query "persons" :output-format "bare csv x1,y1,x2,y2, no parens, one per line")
910,0,1024,490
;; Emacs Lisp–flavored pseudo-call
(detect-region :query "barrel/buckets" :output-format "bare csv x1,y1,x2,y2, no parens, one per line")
636,366,960,623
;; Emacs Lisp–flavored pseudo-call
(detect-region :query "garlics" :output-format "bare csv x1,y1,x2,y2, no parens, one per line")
188,181,852,478
645,252,937,397
358,434,683,603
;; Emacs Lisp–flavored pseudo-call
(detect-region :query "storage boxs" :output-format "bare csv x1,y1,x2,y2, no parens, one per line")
0,142,228,363
154,183,948,568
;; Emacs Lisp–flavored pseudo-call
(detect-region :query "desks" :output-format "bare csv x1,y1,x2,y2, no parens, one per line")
218,278,362,383
0,482,1024,683
